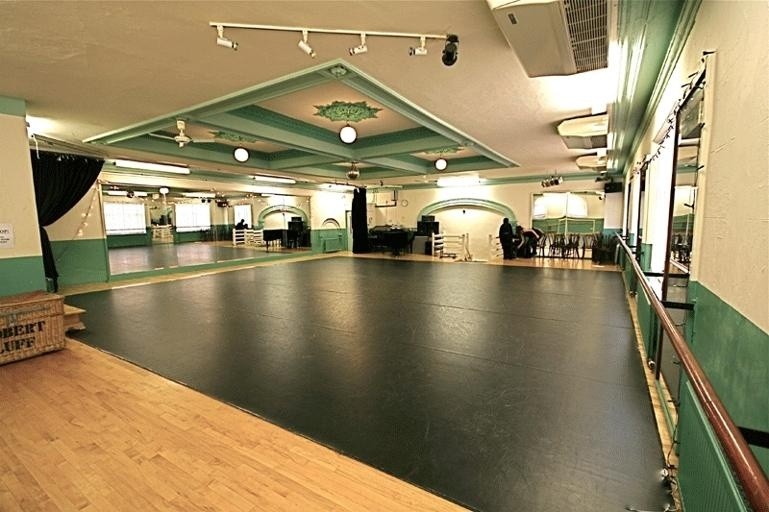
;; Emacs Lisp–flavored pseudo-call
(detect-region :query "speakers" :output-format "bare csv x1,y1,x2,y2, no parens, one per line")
605,182,622,193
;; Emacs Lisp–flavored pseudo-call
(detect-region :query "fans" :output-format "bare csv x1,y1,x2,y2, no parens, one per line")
149,120,215,148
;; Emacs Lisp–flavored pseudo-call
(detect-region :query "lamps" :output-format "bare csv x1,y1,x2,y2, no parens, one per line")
541,175,563,187
210,21,447,59
435,152,448,171
345,161,360,181
340,112,357,143
234,143,249,163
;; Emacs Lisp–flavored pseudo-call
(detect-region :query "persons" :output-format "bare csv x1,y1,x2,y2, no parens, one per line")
243,223,248,229
532,226,545,241
499,217,514,260
515,224,539,258
235,218,244,242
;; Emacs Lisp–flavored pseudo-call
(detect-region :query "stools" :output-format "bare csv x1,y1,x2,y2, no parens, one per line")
65,305,87,332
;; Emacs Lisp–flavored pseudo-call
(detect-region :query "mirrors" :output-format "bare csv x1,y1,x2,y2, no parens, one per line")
623,67,706,412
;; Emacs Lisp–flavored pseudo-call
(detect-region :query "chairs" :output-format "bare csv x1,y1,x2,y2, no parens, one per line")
538,231,595,259
